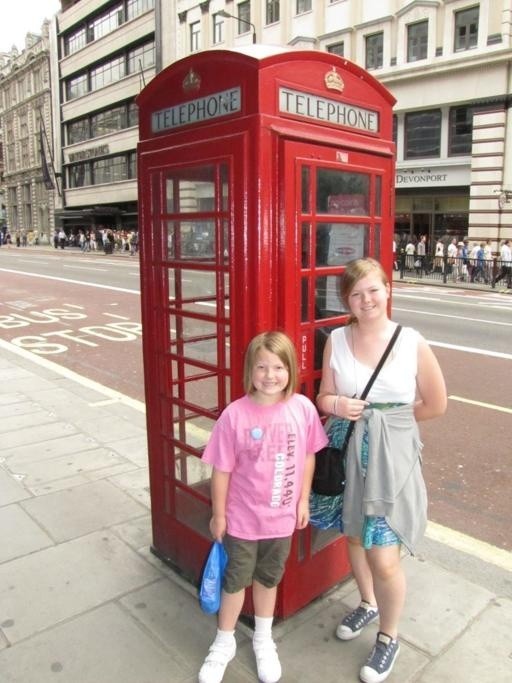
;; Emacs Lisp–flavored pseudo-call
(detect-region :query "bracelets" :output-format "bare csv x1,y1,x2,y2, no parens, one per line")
331,395,339,415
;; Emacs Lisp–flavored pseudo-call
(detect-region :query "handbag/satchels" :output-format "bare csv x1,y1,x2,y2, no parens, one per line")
310,446,347,497
414,259,422,267
199,540,229,616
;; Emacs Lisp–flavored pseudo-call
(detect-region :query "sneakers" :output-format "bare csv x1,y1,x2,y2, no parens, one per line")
335,599,380,641
198,637,237,683
359,630,401,683
252,634,282,683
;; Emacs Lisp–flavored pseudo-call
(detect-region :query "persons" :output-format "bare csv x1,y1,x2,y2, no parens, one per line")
198,330,330,683
316,255,448,683
1,223,139,255
389,229,511,291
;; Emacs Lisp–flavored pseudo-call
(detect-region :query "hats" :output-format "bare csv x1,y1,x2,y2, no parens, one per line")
457,241,464,245
